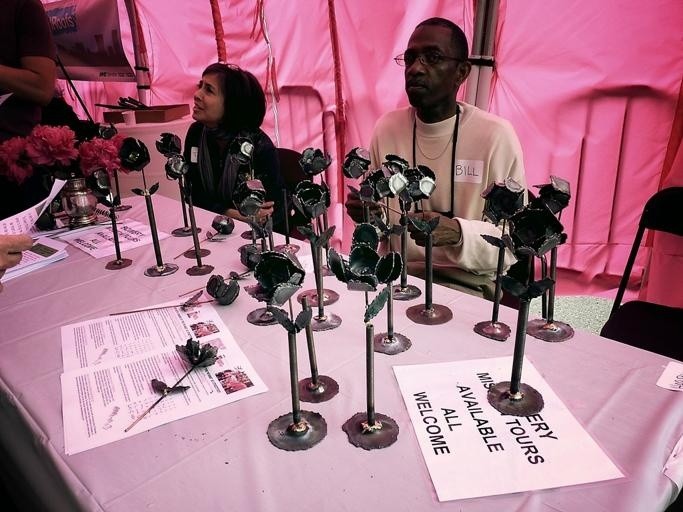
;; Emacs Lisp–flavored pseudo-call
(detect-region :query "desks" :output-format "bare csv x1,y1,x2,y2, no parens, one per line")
0,149,683,188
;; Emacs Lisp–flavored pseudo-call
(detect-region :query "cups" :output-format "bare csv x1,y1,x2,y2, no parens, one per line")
122,110,136,127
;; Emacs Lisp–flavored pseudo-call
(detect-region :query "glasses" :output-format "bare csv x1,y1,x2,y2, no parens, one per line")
394,52,463,66
218,61,240,72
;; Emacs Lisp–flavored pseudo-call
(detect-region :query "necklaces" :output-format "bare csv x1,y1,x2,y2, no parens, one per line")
414,118,456,162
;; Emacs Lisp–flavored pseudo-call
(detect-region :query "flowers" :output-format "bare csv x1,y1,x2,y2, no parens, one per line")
0,123,138,186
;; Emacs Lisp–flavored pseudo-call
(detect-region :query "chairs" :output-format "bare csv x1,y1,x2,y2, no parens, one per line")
599,187,683,363
273,148,318,237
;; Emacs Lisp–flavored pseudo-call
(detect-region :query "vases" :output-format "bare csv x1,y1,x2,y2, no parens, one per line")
57,176,107,227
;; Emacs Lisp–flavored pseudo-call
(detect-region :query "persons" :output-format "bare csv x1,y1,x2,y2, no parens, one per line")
345,16,527,304
0,234,33,294
184,64,285,231
0,0,57,133
